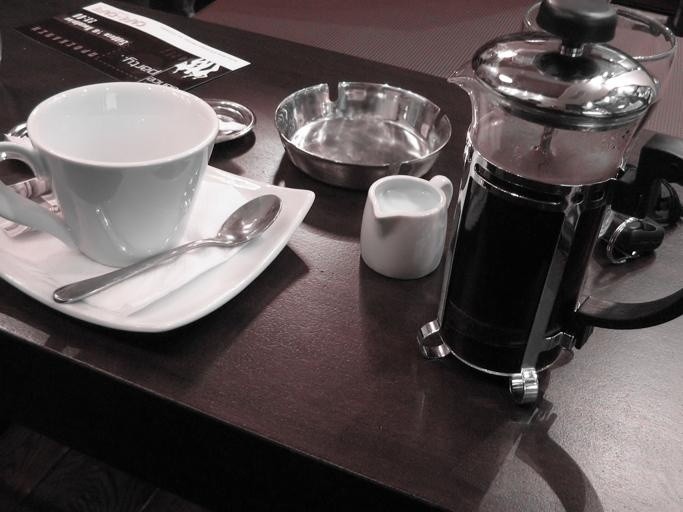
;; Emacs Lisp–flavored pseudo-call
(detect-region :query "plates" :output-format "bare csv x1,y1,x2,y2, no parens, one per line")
0,162,318,334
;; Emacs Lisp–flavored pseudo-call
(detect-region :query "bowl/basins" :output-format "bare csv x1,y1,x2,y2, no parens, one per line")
273,79,453,191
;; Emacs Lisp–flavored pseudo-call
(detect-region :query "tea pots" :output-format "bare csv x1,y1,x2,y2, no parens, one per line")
416,0,683,407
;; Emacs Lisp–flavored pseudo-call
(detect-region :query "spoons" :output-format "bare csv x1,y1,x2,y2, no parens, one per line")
52,191,283,303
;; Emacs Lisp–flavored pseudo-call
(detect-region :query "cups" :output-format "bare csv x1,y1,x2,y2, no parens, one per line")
360,173,455,280
0,80,222,270
519,0,678,166
